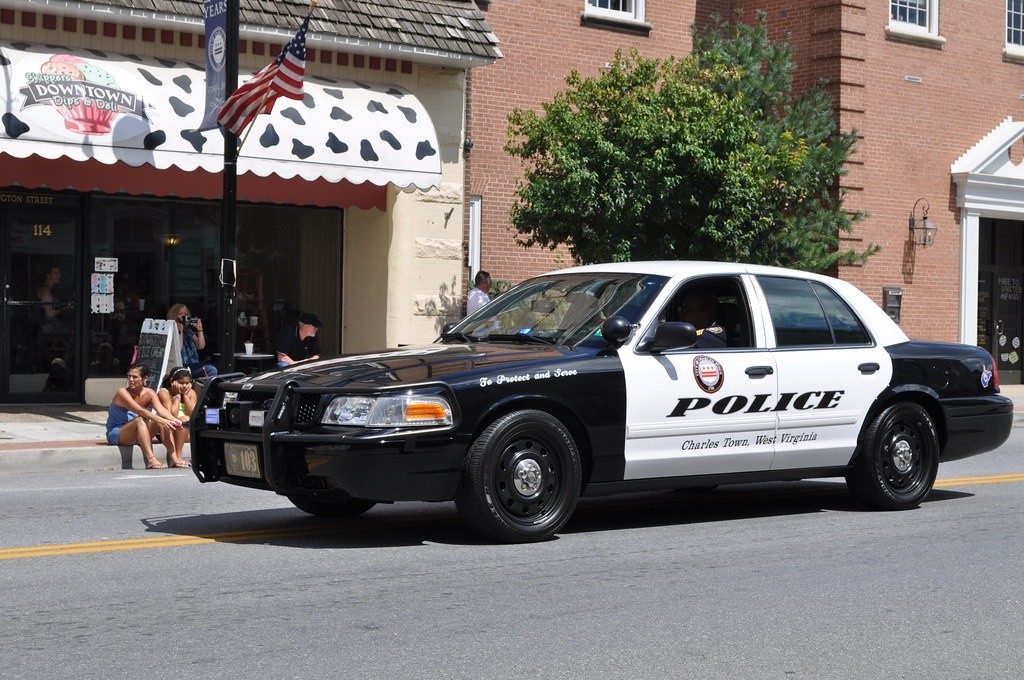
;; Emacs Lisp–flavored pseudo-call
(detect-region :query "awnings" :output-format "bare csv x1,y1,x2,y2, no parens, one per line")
0,35,444,197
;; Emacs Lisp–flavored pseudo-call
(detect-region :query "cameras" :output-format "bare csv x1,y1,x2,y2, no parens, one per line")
182,315,198,324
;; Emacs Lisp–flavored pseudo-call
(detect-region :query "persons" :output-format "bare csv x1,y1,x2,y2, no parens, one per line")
37,265,70,318
48,358,84,404
466,271,492,316
164,303,323,376
91,342,119,372
679,288,728,349
105,363,198,469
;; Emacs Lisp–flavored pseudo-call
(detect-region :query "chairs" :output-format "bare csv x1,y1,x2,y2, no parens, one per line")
714,303,749,348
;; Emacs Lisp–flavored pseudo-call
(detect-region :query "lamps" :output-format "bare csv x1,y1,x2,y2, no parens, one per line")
909,198,937,249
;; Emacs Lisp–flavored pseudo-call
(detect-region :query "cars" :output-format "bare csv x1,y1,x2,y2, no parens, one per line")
189,261,1013,543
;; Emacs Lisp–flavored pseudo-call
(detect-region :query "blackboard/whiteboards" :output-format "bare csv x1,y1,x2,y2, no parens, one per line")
127,318,174,395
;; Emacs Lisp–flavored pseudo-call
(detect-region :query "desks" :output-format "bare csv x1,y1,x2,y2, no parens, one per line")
212,353,274,375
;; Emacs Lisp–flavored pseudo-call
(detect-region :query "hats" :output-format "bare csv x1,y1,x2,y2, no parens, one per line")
299,313,322,328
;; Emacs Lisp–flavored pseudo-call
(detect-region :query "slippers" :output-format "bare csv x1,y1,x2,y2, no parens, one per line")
169,462,190,468
145,463,166,469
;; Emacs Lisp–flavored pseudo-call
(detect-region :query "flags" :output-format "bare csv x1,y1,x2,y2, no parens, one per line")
216,21,307,137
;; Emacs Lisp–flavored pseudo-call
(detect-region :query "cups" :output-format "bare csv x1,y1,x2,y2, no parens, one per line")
245,344,253,355
